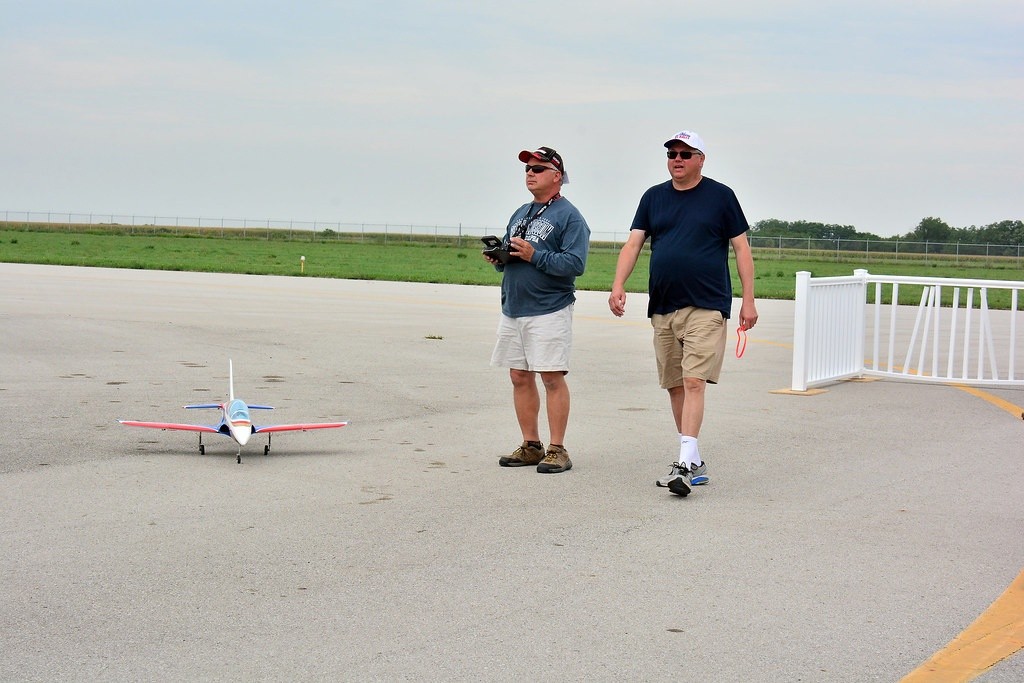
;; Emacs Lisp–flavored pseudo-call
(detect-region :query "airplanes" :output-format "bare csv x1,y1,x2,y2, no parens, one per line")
117,357,347,463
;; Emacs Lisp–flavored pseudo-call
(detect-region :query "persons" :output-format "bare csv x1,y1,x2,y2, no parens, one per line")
481,146,591,474
608,129,759,496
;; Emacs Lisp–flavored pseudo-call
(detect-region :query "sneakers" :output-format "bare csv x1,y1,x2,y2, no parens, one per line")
536,444,572,473
656,461,709,487
498,441,546,467
666,461,691,498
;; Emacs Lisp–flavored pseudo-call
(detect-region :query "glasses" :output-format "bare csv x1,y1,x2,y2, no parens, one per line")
525,165,556,174
667,151,700,160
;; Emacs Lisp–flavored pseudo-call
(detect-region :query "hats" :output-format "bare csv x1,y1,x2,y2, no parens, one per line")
664,131,706,157
519,146,564,175
